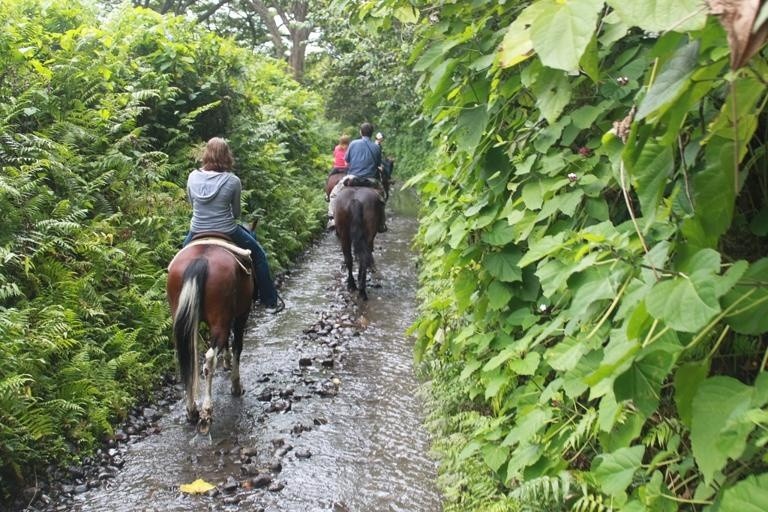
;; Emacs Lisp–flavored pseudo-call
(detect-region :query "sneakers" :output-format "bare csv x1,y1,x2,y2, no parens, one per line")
266,303,278,314
379,224,386,233
327,219,336,229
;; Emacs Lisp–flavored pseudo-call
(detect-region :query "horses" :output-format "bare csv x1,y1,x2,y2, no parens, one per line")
332,177,386,302
381,156,396,181
165,215,261,437
324,170,348,201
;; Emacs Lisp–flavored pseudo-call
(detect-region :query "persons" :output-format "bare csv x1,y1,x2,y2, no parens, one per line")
323,134,349,201
327,123,388,233
182,137,284,314
374,131,385,164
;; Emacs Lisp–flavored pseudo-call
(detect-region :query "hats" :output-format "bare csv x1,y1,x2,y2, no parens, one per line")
375,132,384,139
